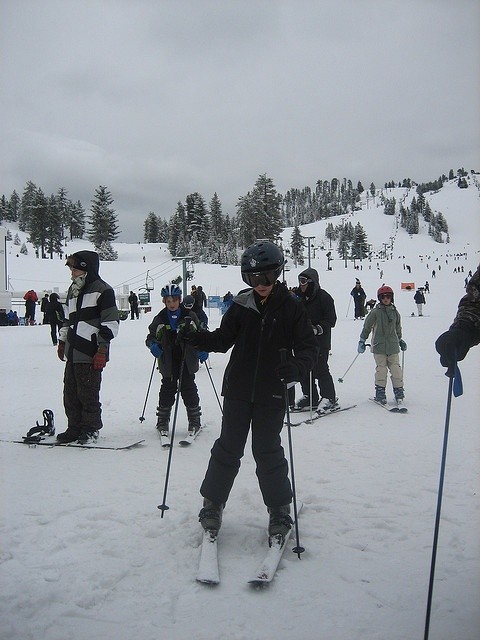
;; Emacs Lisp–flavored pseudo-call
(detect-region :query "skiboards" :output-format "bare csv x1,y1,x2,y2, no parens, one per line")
369,397,408,412
197,499,303,586
156,424,203,447
284,405,357,426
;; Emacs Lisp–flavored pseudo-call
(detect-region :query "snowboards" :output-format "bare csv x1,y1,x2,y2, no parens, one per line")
13,435,145,449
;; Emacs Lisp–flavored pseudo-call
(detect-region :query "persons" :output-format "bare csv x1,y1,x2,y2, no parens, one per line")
379,270,384,279
13,311,20,325
191,286,207,310
191,270,195,279
424,281,430,295
127,290,139,320
0,308,7,325
350,280,367,321
175,294,209,390
293,267,338,414
413,287,427,316
223,294,229,307
434,261,480,368
175,240,321,549
464,277,469,288
432,270,436,278
45,292,65,346
40,293,52,324
357,285,407,404
24,296,36,323
144,283,210,437
185,270,191,282
7,309,17,326
143,255,145,263
286,386,296,411
406,265,410,273
23,289,38,304
56,250,120,445
227,290,233,306
191,285,196,295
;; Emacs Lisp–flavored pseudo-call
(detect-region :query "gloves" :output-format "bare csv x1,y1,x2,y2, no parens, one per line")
57,340,66,362
196,350,209,361
276,359,299,391
399,339,407,352
176,320,197,342
358,338,366,354
90,347,107,371
312,325,323,336
434,320,478,367
148,340,162,358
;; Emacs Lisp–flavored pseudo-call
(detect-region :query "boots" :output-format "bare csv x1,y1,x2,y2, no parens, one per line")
187,406,202,435
157,407,171,433
376,387,387,403
394,387,404,405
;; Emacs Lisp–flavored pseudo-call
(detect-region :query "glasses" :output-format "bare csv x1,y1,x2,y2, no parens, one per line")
67,255,79,267
242,262,285,287
379,294,392,299
299,276,311,285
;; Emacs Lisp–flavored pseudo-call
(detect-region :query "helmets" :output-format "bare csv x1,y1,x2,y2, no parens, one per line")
377,287,394,303
183,295,196,310
242,241,284,285
49,293,60,302
161,284,182,296
71,250,99,282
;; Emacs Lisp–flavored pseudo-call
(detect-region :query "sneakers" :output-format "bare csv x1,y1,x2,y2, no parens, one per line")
200,498,225,532
57,426,80,442
291,395,319,411
77,429,99,445
268,507,293,537
316,398,337,415
